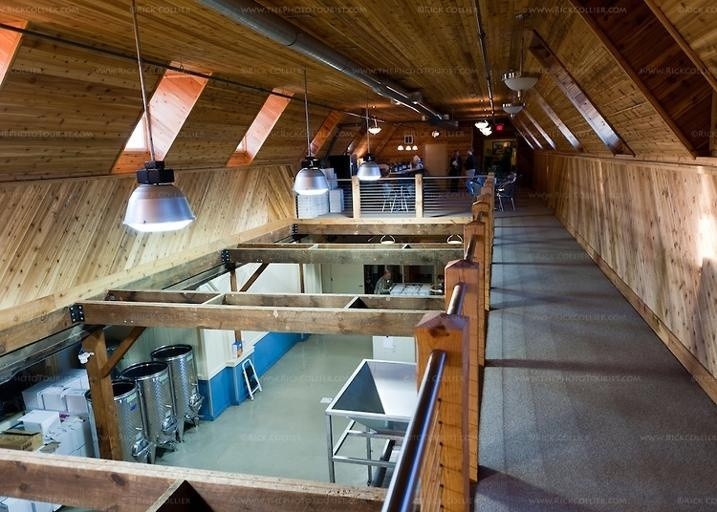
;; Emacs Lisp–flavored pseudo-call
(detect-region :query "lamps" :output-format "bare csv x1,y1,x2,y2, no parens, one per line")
124,0,198,235
480,124,492,132
473,97,489,129
291,63,332,197
380,235,396,244
499,0,543,91
396,134,420,151
502,89,526,114
368,115,383,135
356,92,383,182
447,234,464,245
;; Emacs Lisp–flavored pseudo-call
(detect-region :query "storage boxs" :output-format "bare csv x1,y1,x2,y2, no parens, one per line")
389,283,433,296
0,369,101,512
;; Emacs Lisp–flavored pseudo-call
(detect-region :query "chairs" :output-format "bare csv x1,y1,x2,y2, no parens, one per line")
381,180,416,212
465,164,521,212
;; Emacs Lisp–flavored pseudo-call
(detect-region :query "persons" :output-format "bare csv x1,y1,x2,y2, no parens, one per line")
373,269,392,294
448,148,464,192
378,164,394,198
463,149,476,192
